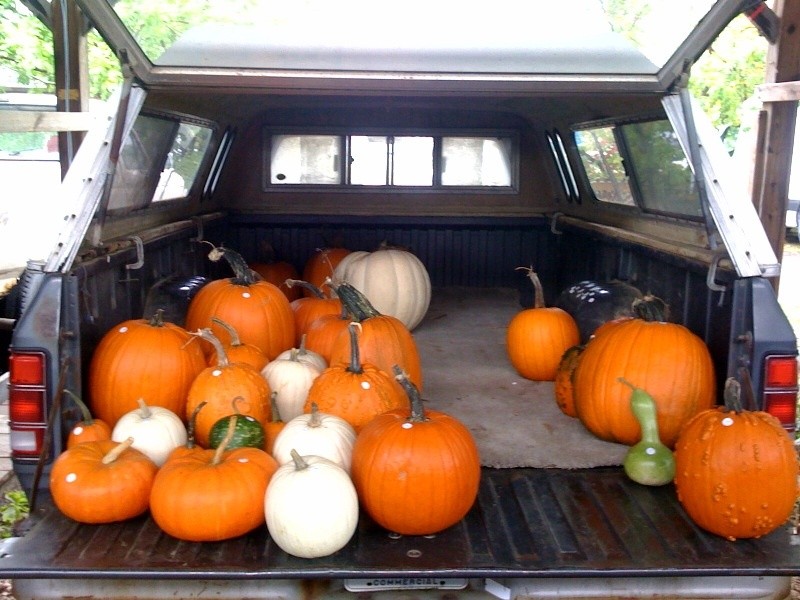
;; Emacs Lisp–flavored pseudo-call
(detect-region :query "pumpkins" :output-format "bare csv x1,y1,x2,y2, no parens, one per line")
507,272,799,541
49,240,479,559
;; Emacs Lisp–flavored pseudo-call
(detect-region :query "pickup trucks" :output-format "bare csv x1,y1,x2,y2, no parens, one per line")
0,0,799,600
